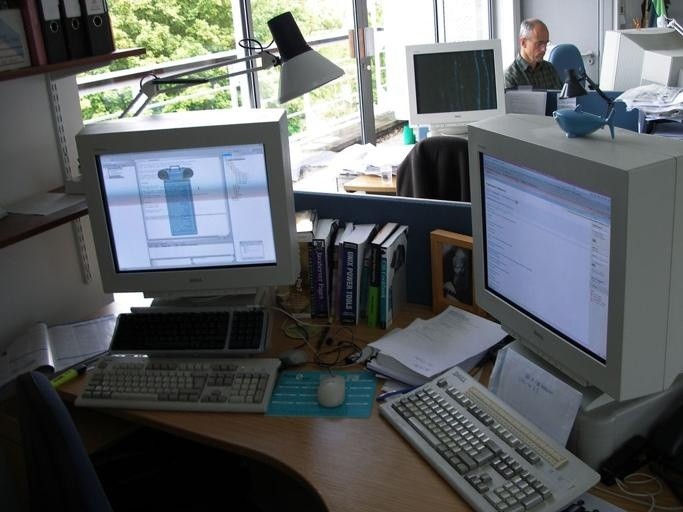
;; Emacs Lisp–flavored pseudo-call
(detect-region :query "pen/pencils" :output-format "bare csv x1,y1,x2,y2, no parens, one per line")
315,317,335,351
50,350,112,389
632,17,641,32
377,385,421,402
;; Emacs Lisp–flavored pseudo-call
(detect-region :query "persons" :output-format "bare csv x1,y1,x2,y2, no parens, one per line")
503,18,563,93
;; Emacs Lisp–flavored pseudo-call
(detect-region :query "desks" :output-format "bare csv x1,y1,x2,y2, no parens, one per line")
55,304,681,512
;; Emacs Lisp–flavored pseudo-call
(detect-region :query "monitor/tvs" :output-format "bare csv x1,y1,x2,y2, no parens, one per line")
467,114,683,402
405,39,506,135
74,109,299,298
598,28,683,92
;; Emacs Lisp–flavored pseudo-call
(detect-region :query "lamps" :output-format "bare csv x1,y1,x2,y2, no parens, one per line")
558,65,613,111
119,17,346,115
657,14,683,37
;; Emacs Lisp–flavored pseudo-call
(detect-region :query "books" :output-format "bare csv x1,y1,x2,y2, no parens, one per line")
293,208,409,329
364,302,510,388
328,141,415,177
0,313,118,386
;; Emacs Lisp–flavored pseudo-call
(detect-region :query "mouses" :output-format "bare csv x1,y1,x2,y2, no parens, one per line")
318,376,347,407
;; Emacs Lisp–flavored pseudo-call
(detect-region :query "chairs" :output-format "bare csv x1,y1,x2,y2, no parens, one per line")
550,43,587,92
395,136,470,201
19,368,115,511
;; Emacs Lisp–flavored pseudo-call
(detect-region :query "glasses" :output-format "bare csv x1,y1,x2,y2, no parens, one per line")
526,38,551,48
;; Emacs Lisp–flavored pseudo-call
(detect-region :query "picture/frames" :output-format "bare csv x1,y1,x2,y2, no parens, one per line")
429,228,487,320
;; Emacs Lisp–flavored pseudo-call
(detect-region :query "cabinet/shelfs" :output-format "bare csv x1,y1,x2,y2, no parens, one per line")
0,48,153,253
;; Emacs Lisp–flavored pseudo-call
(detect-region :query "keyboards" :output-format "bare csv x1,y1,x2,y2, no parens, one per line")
380,367,601,512
73,357,281,414
110,308,266,357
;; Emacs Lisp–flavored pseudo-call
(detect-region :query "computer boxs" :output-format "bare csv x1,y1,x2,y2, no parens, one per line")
498,338,683,467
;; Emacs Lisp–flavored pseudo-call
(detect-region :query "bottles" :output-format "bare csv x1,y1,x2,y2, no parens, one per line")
157,166,197,237
